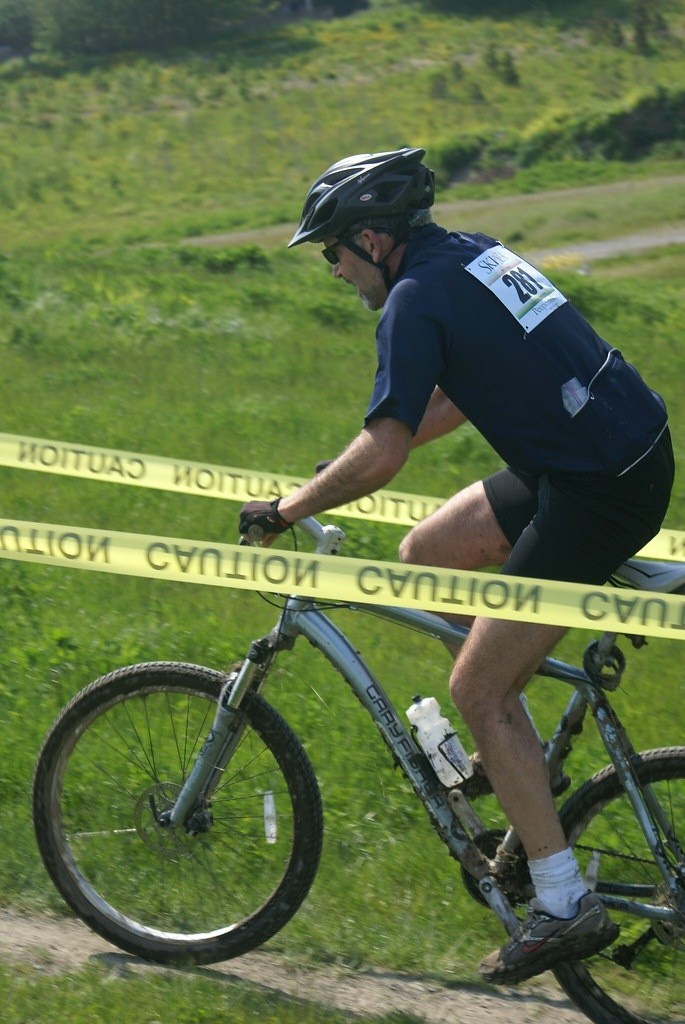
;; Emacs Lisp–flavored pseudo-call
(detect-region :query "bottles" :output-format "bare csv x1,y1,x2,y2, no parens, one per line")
405,695,473,787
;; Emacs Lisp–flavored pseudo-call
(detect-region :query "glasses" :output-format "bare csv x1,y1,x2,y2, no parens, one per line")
321,231,361,265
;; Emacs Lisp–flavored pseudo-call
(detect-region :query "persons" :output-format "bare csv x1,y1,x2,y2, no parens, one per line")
238,148,675,984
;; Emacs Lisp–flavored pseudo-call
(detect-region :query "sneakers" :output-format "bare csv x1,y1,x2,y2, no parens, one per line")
451,741,570,799
477,889,620,987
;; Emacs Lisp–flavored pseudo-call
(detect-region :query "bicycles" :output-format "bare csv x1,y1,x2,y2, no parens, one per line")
29,491,685,1024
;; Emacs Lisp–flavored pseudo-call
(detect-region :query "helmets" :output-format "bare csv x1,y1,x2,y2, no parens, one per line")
287,148,437,253
